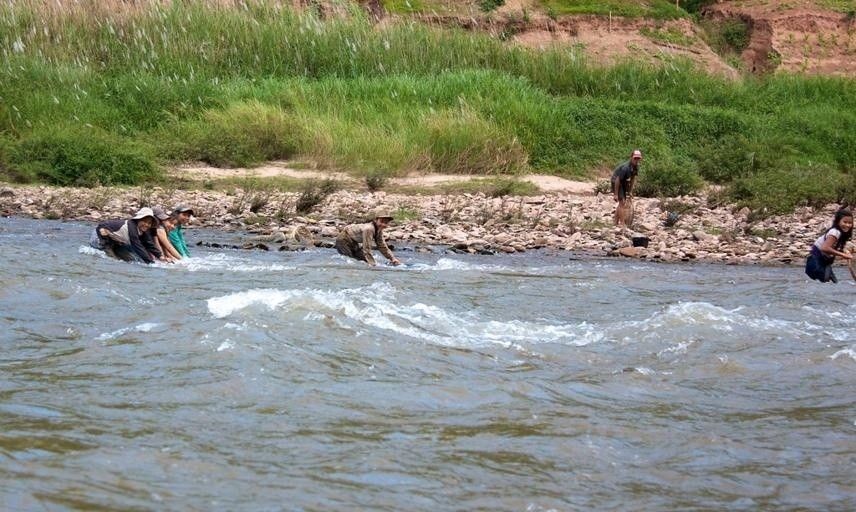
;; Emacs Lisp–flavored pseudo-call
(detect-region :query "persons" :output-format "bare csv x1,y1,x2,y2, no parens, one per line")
96,202,194,264
336,210,400,266
806,209,856,283
611,149,642,226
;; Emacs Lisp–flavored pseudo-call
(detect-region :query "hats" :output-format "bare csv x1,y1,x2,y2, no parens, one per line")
131,201,194,229
632,151,643,159
377,211,394,221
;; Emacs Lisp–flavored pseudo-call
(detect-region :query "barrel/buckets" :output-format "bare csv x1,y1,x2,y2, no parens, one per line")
632,237,650,247
